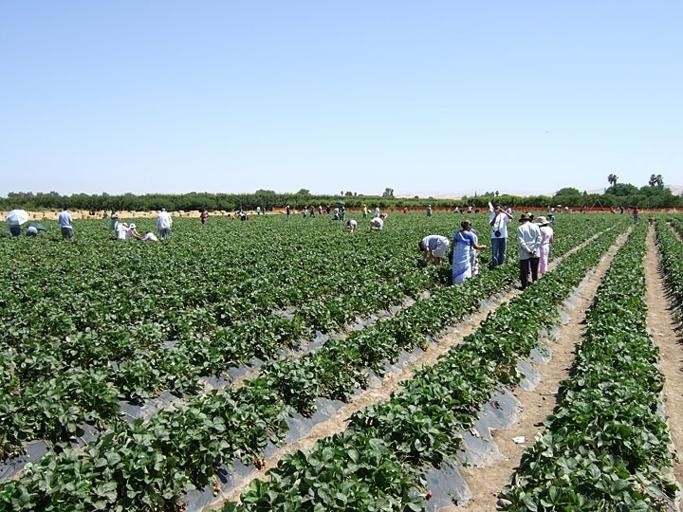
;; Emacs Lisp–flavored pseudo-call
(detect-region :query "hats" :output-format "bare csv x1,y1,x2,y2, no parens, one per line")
518,213,531,222
534,216,549,226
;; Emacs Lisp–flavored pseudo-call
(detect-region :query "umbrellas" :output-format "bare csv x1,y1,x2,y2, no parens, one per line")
7,210,29,226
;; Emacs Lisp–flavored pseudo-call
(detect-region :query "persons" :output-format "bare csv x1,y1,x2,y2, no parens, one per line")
11,227,20,236
233,205,265,221
347,204,387,230
199,207,208,225
427,204,432,217
487,203,513,270
403,205,408,214
285,204,290,216
610,204,638,224
556,204,569,214
516,211,554,289
418,218,488,285
27,220,48,237
57,206,75,240
301,205,346,222
102,205,172,243
453,204,482,213
